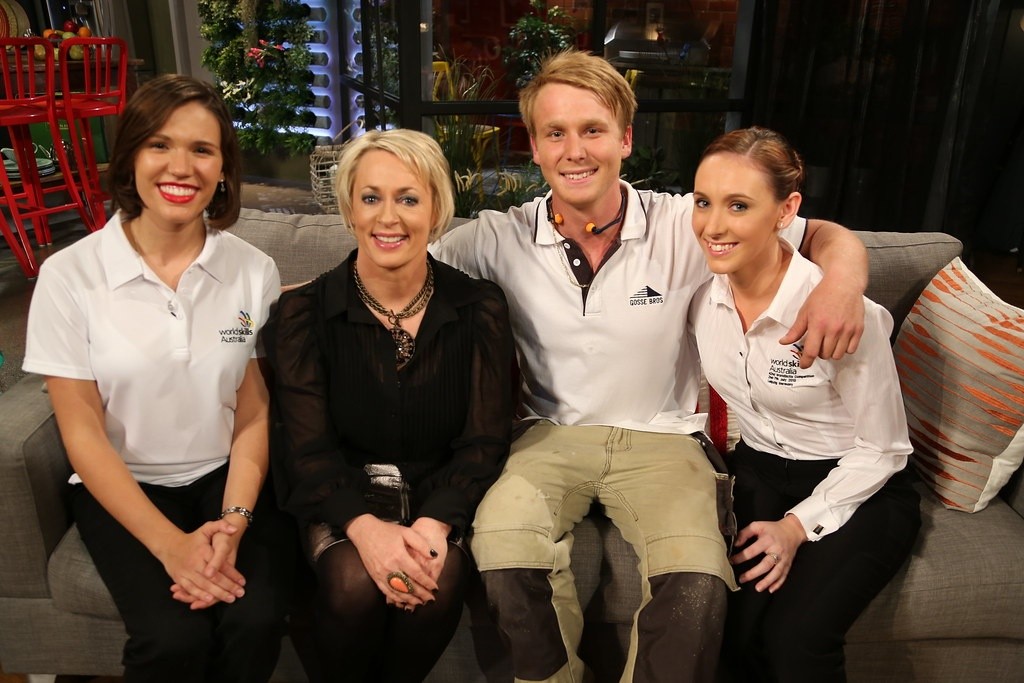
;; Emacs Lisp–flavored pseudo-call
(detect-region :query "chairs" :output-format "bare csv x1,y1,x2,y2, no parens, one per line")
433,59,503,201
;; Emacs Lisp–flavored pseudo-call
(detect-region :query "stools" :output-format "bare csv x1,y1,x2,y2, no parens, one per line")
24,36,128,246
0,37,96,276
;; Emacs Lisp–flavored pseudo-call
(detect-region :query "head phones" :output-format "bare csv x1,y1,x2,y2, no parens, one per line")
547,191,625,234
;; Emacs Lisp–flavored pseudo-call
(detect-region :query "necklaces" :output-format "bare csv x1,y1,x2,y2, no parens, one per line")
352,260,434,370
547,188,629,288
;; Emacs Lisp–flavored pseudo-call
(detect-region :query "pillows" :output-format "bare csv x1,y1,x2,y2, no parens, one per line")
894,255,1024,514
693,356,741,451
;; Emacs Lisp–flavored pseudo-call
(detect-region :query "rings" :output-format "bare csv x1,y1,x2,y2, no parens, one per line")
385,571,414,594
766,552,777,565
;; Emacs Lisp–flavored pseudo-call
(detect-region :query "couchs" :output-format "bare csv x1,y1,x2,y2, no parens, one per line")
0,206,1024,683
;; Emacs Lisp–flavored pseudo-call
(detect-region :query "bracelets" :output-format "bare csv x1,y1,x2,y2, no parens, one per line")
218,506,254,522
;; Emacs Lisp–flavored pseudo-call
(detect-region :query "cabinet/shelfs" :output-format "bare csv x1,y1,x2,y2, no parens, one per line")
0,58,144,194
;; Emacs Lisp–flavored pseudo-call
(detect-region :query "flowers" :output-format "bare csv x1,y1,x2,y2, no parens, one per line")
220,78,263,113
246,41,284,67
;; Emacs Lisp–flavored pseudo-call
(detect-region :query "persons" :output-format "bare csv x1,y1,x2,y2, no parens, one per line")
686,125,922,683
256,129,526,683
277,51,870,683
17,71,281,683
